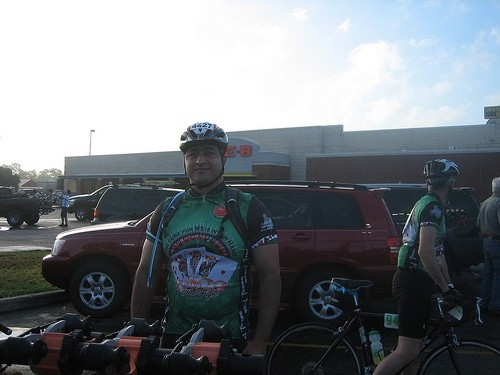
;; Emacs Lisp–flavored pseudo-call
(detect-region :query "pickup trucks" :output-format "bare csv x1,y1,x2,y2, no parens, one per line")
0,186,56,226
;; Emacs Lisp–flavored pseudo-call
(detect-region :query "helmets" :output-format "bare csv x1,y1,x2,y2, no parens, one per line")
179,122,228,151
424,158,459,177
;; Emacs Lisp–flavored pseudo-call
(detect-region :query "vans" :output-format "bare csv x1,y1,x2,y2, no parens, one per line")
354,183,483,274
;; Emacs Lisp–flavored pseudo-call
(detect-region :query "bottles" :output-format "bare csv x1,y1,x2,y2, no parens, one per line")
368,329,384,365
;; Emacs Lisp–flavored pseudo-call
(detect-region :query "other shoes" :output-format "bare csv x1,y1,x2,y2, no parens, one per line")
59,223,68,227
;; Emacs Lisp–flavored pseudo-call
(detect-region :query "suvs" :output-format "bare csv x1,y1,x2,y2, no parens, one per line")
68,185,141,222
41,181,404,316
91,186,185,225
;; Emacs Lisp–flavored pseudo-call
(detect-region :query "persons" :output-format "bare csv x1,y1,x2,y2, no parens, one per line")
374,160,462,375
476,178,499,316
30,189,56,206
131,123,282,362
58,189,69,226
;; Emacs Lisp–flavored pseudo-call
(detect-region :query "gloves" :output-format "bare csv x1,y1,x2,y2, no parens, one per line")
442,285,462,298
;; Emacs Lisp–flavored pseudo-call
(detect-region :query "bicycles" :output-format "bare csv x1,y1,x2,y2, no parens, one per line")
266,277,499,375
